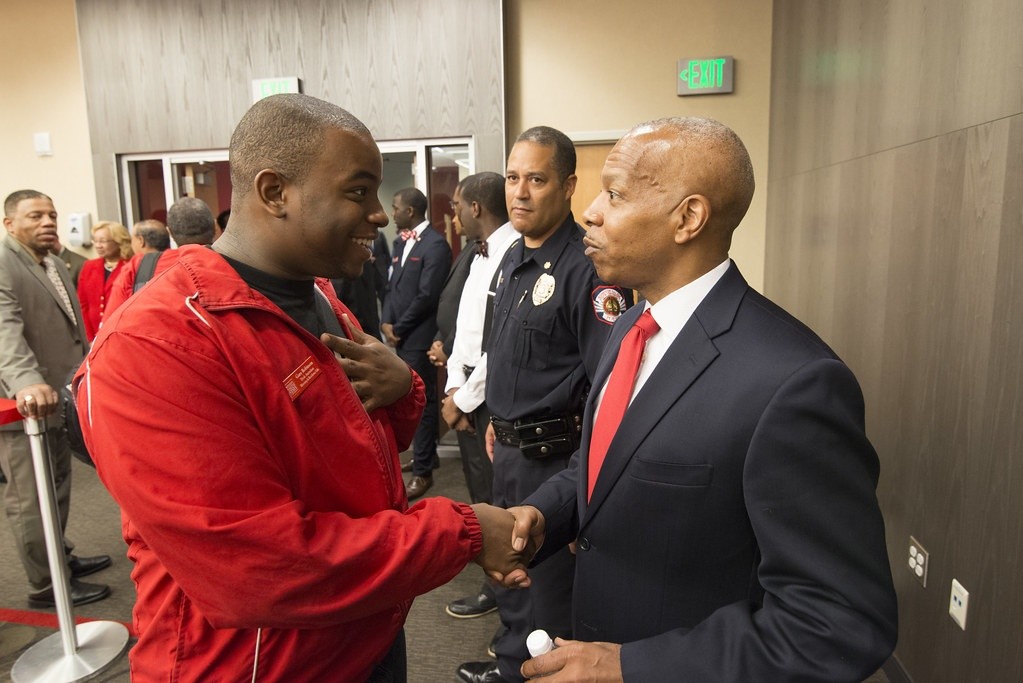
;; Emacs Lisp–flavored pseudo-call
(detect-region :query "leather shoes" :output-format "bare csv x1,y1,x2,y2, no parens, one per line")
27,580,110,608
406,471,433,500
402,455,440,472
446,592,498,618
456,662,502,683
488,627,508,658
69,554,111,580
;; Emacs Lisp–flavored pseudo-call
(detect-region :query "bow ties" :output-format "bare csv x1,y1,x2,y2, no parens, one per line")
477,243,488,257
399,229,416,241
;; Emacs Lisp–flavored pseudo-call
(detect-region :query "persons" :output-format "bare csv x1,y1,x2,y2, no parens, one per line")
486,116,899,683
50,197,230,351
70,93,537,683
428,125,635,683
336,186,453,501
0,190,112,609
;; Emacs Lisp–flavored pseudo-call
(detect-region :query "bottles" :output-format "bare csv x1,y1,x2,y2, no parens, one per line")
526,629,560,680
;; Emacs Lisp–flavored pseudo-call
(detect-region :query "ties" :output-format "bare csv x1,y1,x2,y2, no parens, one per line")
42,256,79,328
587,308,660,507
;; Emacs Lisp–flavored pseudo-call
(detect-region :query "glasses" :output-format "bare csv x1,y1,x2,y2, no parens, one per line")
92,238,114,244
